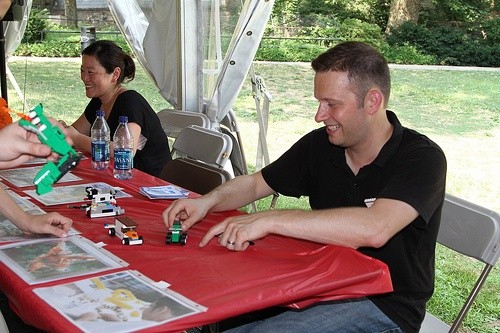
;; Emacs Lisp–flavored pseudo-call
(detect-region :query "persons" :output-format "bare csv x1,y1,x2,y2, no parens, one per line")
74,295,191,333
0,117,73,169
0,184,73,237
58,40,173,181
163,42,447,333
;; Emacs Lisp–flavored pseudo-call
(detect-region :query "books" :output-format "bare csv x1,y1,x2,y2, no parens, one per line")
140,186,189,199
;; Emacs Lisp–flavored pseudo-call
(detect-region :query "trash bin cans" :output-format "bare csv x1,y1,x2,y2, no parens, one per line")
82,26,95,50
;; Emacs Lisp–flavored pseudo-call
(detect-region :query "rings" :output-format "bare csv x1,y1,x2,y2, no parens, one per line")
227,240,235,245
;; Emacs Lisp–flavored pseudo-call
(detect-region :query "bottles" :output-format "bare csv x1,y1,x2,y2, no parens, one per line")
91,110,110,171
113,116,133,179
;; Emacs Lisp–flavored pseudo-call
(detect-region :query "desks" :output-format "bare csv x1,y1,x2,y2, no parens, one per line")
0,147,395,333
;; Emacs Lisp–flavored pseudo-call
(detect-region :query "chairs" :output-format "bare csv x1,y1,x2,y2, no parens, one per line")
170,125,234,168
156,108,210,158
419,191,500,333
158,156,232,196
